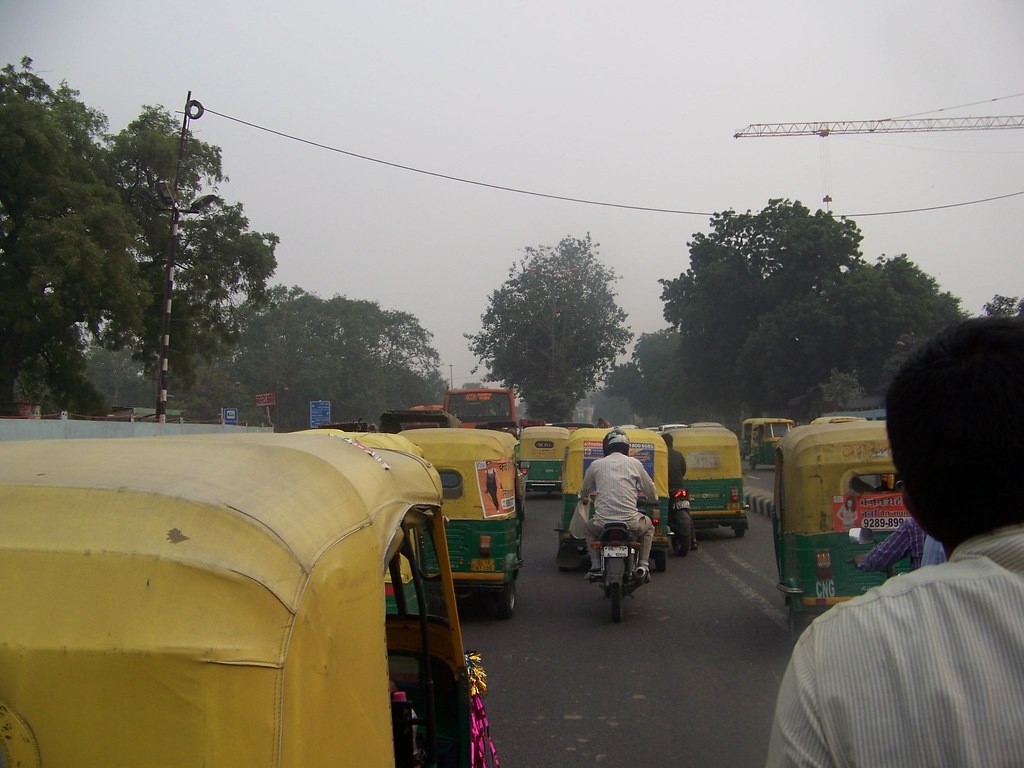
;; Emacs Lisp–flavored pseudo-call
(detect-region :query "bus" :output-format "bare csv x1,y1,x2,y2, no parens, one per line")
443,384,518,436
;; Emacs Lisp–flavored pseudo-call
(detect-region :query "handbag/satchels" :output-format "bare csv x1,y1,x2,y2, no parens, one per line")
568,499,590,540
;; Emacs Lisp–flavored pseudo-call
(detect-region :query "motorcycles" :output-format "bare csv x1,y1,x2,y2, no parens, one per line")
514,426,570,494
741,418,797,472
1,433,474,768
661,427,750,537
553,427,675,574
582,499,660,624
396,427,533,621
772,420,912,645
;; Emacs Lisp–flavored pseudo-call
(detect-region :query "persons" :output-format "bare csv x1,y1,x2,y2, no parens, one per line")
664,434,699,550
854,516,948,570
763,318,1023,768
578,431,662,585
838,496,858,530
484,461,503,514
596,416,614,429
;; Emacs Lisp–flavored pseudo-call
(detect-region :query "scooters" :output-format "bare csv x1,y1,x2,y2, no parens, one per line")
667,485,694,559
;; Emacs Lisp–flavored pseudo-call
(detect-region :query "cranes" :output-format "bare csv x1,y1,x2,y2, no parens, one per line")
731,88,1024,140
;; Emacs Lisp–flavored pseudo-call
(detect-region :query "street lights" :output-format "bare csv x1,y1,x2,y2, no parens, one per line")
155,180,220,424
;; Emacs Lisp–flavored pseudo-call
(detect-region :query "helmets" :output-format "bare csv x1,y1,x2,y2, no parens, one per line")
603,430,630,456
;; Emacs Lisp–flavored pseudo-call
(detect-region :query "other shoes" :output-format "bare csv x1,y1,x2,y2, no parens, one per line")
646,569,651,584
583,568,603,578
691,540,698,550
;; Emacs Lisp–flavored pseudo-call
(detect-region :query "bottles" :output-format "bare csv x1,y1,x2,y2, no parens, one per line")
394,691,419,768
